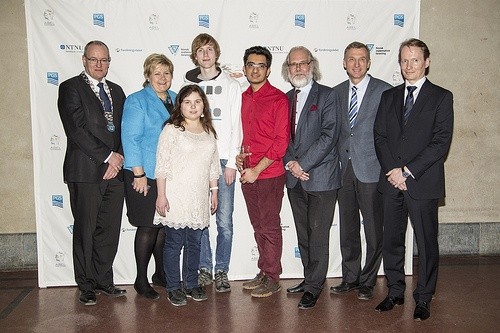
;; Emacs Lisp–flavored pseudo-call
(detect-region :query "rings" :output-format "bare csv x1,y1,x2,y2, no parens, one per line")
235,162,237,165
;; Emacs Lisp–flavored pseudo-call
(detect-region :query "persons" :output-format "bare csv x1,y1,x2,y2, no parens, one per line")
120,53,178,300
282,46,341,310
181,33,243,293
374,39,454,322
57,41,126,306
330,41,396,300
152,85,223,307
235,46,290,298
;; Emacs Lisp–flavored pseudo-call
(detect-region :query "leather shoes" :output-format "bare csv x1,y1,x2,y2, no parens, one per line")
357,286,373,300
330,282,358,292
95,284,127,297
375,295,404,311
287,278,305,294
79,289,97,305
297,287,324,309
413,301,430,321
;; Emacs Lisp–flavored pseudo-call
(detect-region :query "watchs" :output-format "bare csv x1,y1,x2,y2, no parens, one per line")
402,167,409,178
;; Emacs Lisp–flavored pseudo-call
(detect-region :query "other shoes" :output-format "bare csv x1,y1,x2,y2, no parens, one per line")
134,282,161,300
152,273,167,287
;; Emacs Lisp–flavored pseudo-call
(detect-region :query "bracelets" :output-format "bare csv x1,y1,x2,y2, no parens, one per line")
134,173,146,178
210,187,219,191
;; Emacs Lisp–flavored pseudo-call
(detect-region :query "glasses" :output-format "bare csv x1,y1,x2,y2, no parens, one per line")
244,63,269,70
287,60,313,68
84,54,111,65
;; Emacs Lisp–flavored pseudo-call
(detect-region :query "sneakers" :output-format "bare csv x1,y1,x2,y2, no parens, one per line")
242,270,266,289
214,269,231,292
251,272,283,297
197,268,213,287
167,289,188,306
186,287,208,301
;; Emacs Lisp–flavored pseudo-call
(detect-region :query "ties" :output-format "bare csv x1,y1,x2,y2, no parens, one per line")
291,90,301,143
97,82,111,112
348,86,359,130
403,86,417,128
